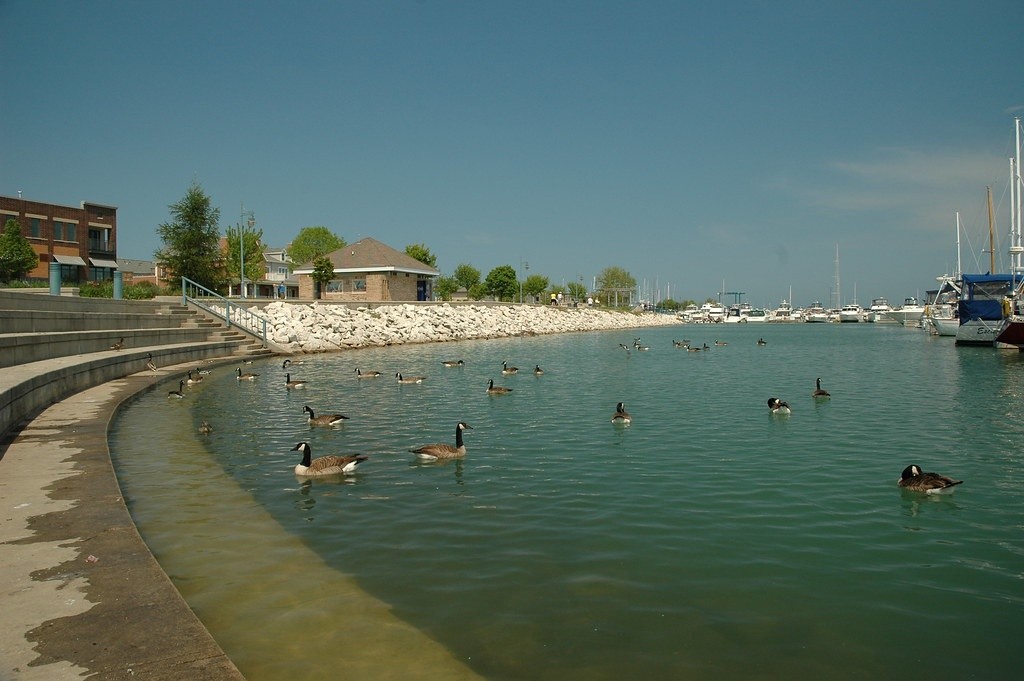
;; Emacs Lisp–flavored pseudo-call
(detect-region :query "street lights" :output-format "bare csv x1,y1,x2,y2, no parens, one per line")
575,272,585,302
518,257,530,304
239,202,257,300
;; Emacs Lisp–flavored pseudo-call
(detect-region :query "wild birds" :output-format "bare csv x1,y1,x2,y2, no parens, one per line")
109,337,965,497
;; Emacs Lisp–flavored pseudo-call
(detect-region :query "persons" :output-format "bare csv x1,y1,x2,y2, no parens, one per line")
587,297,600,309
550,291,563,306
278,281,286,300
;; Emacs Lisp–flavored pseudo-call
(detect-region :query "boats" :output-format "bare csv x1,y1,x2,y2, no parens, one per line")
676,115,1024,354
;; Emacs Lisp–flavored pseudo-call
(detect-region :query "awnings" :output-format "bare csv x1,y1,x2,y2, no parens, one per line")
88,257,119,268
53,254,86,266
